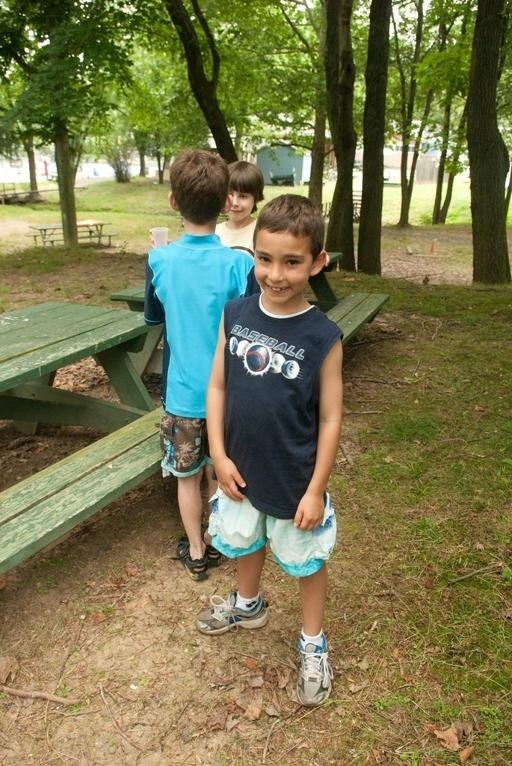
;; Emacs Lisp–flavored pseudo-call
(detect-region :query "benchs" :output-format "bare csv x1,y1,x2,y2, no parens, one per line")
0,404,221,576
324,292,391,347
24,219,119,246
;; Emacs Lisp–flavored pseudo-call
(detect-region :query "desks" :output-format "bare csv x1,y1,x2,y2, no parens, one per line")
110,236,343,379
0,299,160,434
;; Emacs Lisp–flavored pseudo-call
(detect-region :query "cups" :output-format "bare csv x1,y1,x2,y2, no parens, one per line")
151,227,168,248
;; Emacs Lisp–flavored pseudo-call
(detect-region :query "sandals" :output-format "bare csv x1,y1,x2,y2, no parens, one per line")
171,536,208,581
201,522,225,566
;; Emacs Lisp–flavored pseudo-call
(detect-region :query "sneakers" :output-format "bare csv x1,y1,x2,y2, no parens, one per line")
196,592,270,635
296,632,334,707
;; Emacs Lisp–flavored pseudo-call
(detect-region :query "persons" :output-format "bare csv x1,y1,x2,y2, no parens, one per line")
194,194,344,708
212,161,264,261
143,147,256,581
